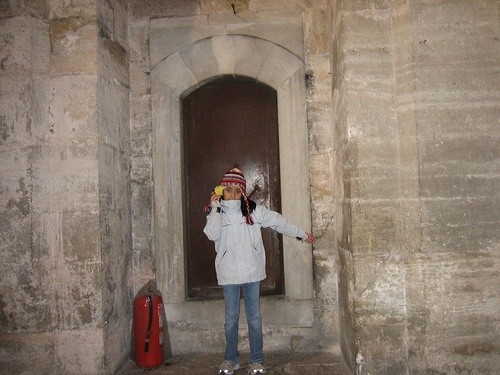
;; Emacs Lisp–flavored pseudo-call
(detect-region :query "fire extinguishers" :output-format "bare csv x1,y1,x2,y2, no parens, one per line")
133,279,166,369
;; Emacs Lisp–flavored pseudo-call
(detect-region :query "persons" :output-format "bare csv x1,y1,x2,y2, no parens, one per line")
203,163,315,375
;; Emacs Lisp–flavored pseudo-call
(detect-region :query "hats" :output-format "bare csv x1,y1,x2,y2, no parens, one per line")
202,163,254,225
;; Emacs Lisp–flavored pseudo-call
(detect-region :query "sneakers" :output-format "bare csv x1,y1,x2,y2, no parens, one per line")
247,362,266,375
218,360,240,375
